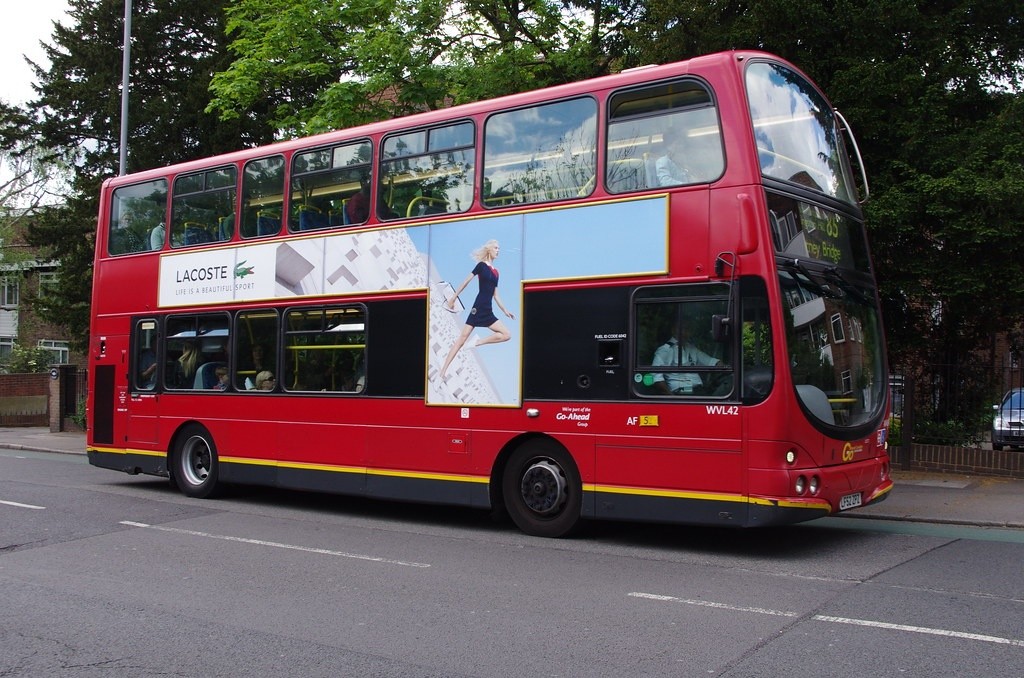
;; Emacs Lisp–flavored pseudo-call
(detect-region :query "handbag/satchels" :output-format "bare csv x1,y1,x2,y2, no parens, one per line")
441,282,465,313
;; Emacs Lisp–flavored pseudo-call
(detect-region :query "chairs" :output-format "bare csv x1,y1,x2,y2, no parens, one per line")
138,349,279,391
145,151,662,253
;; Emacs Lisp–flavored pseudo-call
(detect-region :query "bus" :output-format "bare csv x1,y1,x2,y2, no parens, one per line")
88,50,896,538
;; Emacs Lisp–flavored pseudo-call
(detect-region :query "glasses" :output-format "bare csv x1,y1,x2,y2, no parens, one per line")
262,376,276,382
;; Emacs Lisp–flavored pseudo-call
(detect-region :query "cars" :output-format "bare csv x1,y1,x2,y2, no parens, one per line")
991,386,1024,451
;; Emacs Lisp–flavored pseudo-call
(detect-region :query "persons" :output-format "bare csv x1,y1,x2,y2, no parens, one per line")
223,198,256,239
650,313,731,395
439,239,515,378
139,334,388,395
109,207,185,256
655,126,709,186
348,171,392,224
